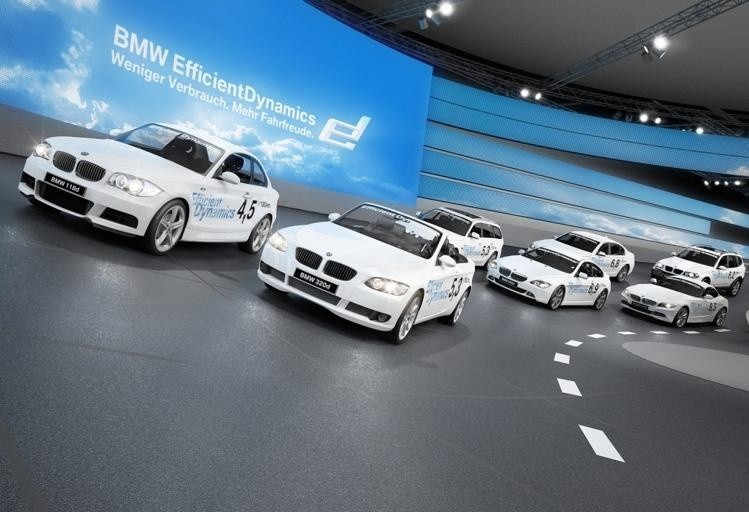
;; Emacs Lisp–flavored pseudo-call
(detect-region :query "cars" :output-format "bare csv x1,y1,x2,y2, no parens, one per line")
527,229,636,280
485,238,611,312
18,122,283,252
412,201,503,267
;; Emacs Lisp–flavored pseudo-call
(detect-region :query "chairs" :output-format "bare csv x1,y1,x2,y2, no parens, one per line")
224,154,246,175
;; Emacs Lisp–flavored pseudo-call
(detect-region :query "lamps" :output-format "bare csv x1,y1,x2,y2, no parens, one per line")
639,30,672,66
417,14,441,29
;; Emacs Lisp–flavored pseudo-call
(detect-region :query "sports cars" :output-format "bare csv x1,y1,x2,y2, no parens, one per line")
253,201,476,344
619,275,730,329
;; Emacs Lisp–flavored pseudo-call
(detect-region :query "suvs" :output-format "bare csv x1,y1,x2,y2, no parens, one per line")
649,243,746,296
